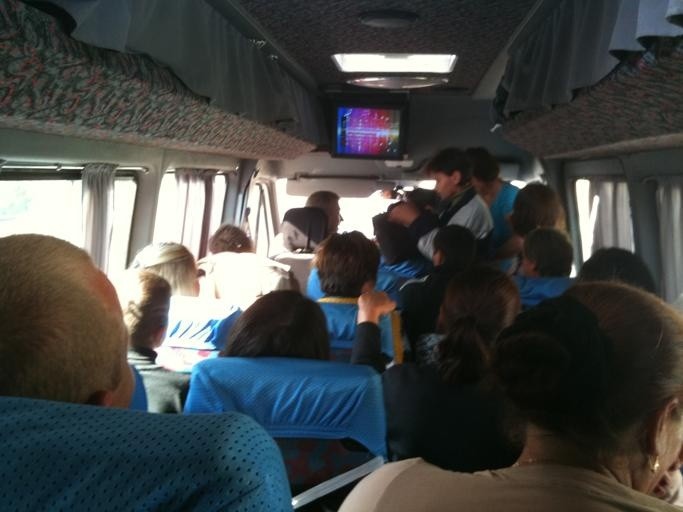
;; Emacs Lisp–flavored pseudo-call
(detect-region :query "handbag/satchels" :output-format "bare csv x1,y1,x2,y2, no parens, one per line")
372,212,411,266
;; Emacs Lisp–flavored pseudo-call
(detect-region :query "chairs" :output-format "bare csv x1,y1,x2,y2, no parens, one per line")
0,207,415,511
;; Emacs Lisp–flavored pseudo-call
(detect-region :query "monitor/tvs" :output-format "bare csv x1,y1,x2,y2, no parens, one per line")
330,99,406,159
